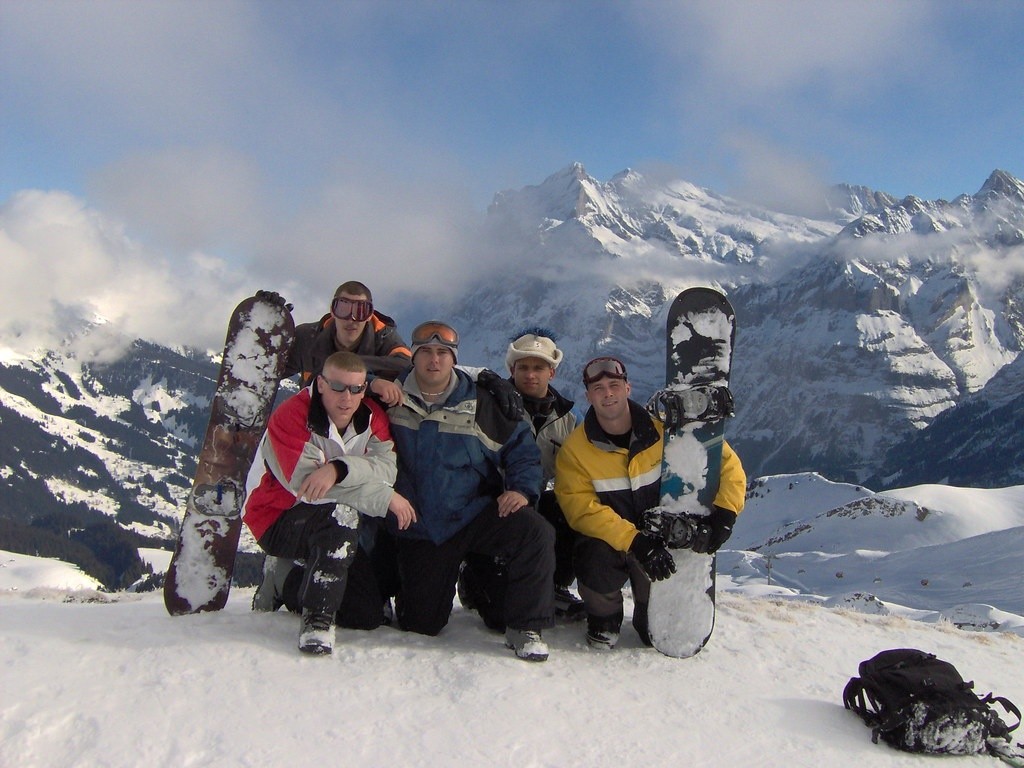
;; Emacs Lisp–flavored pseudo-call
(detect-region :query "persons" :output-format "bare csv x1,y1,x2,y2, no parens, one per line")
451,324,591,634
240,352,417,656
363,320,555,662
553,357,747,651
253,280,412,625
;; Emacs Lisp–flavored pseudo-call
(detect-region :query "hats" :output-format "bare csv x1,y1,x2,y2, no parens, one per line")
409,318,459,365
505,329,562,368
583,356,629,383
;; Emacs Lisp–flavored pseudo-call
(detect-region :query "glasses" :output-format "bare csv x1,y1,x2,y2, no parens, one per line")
410,321,460,349
319,374,367,394
330,296,374,323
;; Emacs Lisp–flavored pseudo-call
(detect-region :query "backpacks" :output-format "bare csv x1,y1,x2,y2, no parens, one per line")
842,649,1022,756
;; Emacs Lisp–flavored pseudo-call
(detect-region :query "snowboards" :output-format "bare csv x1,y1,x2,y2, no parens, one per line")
647,287,737,659
162,295,296,614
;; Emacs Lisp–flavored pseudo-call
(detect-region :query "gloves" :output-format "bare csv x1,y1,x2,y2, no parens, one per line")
633,536,678,582
640,510,696,550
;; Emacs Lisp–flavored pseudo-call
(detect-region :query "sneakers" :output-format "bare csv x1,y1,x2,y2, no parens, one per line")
505,627,549,661
382,599,393,624
457,562,474,610
552,584,586,620
633,608,653,647
252,554,296,612
298,606,336,653
586,618,623,651
